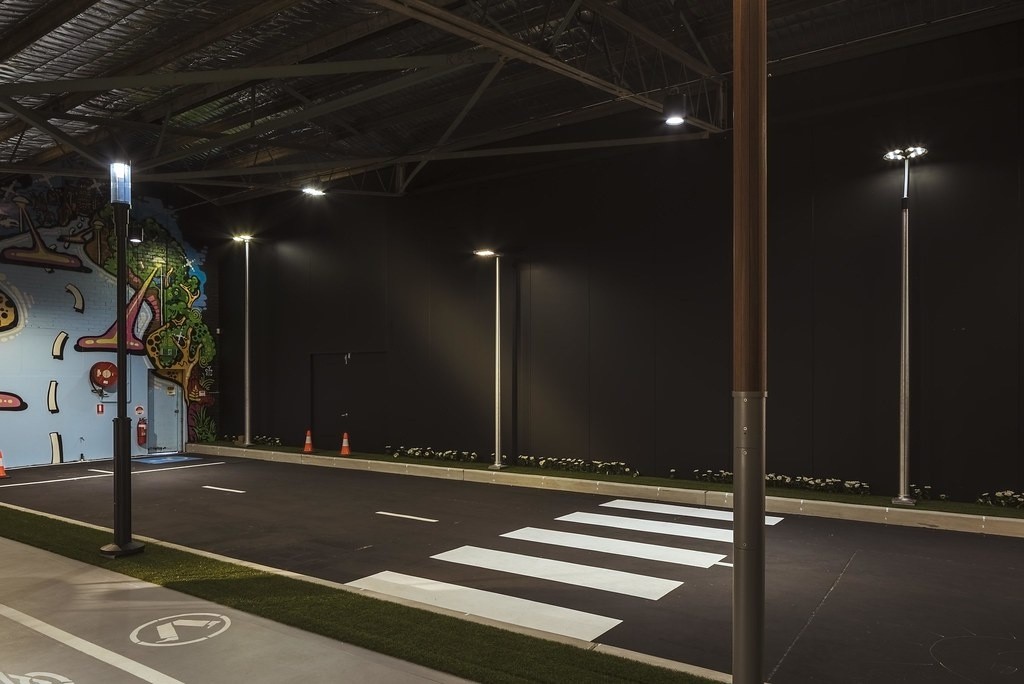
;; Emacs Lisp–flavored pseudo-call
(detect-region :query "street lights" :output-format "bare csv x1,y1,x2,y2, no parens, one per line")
473,249,508,470
882,146,929,506
233,234,255,446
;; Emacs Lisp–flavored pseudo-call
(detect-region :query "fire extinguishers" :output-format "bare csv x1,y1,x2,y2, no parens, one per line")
137,417,147,445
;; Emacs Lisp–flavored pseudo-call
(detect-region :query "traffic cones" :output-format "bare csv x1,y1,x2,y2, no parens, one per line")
341,432,352,456
0,452,11,478
302,429,315,454
